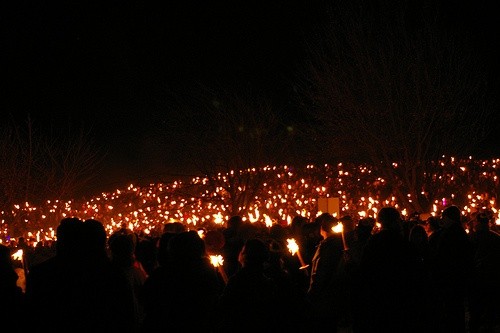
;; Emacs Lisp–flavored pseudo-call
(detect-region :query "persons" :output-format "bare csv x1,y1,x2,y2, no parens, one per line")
0,205,500,333
